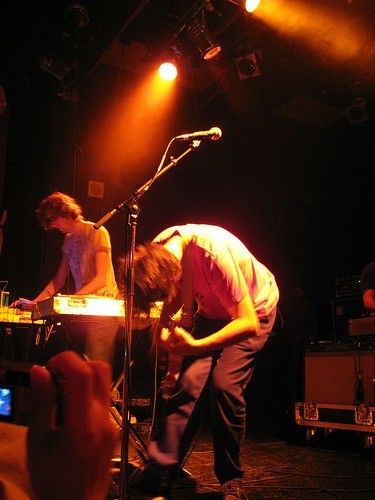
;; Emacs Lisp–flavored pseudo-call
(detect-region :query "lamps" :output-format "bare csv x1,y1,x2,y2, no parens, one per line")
228,0,261,13
234,48,267,82
157,43,192,81
344,85,369,126
184,17,223,61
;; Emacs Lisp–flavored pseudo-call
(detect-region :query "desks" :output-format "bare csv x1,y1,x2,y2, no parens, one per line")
304,350,375,406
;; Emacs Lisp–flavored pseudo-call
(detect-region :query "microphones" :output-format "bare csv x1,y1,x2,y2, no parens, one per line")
176,127,224,142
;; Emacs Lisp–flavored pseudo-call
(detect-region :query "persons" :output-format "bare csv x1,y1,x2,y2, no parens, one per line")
118,224,280,500
0,351,122,500
8,192,120,362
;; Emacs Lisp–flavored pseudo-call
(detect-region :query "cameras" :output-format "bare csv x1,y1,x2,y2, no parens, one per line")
0,359,62,431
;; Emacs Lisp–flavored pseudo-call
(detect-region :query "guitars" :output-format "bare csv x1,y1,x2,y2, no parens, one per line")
160,318,196,390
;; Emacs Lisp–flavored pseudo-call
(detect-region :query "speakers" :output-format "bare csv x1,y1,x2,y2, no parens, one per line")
303,351,375,407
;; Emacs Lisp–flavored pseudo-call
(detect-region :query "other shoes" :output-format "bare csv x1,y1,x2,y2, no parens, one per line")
219,479,248,500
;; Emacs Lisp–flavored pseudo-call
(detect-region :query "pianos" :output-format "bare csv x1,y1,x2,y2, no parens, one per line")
0,292,185,464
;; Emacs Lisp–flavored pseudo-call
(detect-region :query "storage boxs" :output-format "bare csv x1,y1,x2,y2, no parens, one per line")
330,295,372,350
335,275,360,298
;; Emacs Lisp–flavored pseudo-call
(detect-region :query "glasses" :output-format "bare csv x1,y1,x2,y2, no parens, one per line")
45,213,57,225
